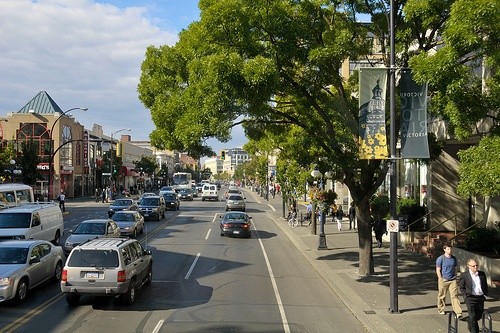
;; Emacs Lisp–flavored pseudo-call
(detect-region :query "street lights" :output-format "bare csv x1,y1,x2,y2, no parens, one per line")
5,159,22,183
312,170,334,250
110,128,131,200
49,107,88,202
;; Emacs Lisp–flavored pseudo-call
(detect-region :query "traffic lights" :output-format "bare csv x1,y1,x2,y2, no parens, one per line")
112,165,117,175
221,151,225,160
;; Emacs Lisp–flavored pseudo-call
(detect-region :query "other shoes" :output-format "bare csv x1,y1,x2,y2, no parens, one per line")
439,310,445,315
456,314,463,318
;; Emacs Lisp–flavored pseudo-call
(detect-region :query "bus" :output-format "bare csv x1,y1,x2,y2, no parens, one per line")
173,172,192,190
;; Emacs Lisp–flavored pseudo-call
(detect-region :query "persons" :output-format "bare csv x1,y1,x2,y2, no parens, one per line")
95,184,116,203
286,196,297,221
347,202,356,231
122,188,132,198
56,191,65,211
315,203,337,219
269,183,281,198
374,214,387,248
435,244,463,320
336,205,343,231
307,201,313,225
460,258,489,333
136,183,145,195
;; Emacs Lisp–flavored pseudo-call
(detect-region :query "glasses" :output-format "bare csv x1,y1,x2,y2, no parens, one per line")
470,265,478,267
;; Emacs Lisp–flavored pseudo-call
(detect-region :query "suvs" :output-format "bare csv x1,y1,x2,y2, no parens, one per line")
138,196,166,221
61,236,153,306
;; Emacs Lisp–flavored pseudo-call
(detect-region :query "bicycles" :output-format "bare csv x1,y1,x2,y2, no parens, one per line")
288,210,298,228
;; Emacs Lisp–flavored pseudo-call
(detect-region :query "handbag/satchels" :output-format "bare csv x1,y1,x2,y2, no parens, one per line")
57,195,60,201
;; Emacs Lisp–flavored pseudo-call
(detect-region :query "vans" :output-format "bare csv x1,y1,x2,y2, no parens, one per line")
0,183,34,211
0,202,64,246
202,184,219,201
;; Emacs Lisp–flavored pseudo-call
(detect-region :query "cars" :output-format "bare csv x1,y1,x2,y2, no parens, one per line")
110,211,144,238
64,219,121,256
159,186,181,211
220,212,252,238
226,195,246,211
108,193,156,218
226,181,243,195
0,239,66,306
176,180,210,201
